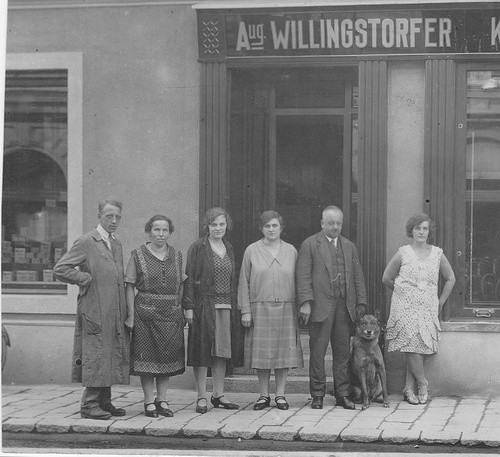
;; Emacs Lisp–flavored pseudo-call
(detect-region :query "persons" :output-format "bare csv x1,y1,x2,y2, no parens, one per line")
125,215,185,417
295,205,366,409
382,213,456,404
236,211,299,410
184,207,244,413
54,200,131,419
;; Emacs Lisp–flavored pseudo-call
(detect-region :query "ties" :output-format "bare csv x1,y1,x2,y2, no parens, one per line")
330,239,335,246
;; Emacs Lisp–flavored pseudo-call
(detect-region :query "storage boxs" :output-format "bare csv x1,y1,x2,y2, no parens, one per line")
2,212,67,282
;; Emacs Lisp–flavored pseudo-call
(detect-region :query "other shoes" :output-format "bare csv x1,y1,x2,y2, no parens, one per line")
101,404,126,416
210,395,239,409
311,396,323,409
80,406,112,420
144,402,158,418
402,388,419,404
254,396,271,410
336,397,355,409
275,396,289,410
196,398,207,414
416,380,428,404
154,398,173,417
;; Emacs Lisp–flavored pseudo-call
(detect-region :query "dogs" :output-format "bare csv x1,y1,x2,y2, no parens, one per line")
348,309,390,409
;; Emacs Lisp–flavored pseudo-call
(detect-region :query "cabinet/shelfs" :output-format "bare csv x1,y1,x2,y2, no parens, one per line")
2,241,64,281
